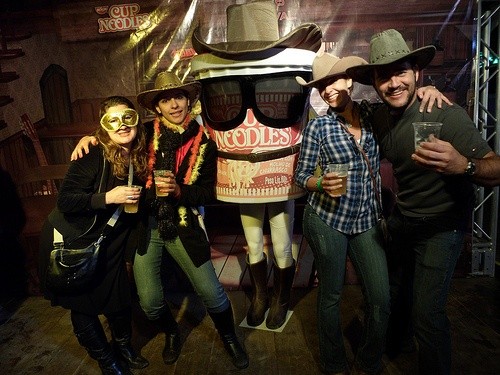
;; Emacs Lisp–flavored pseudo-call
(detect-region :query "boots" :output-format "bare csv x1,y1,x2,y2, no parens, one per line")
207,300,249,369
266,257,296,329
245,252,270,327
72,319,135,375
104,309,149,369
149,307,181,364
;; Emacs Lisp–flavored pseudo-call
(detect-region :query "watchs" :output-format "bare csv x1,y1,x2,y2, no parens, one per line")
463,160,476,177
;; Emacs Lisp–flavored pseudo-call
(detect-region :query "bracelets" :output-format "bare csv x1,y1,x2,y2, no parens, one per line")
317,177,324,193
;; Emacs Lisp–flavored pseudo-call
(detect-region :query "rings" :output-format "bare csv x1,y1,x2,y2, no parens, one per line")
127,195,132,200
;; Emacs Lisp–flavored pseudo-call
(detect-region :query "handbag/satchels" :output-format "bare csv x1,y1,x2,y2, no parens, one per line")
48,243,100,308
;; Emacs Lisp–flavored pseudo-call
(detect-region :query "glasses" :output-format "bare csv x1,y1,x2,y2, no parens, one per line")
199,71,310,131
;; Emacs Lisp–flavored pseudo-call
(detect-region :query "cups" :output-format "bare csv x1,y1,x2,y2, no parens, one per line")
189,48,316,204
329,165,348,196
413,121,443,164
154,171,171,197
124,185,142,214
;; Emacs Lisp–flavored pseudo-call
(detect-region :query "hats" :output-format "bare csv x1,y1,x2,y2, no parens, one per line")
192,2,322,54
296,51,369,88
136,71,202,109
345,28,436,86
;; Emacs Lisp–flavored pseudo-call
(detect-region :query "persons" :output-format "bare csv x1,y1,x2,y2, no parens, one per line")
48,96,150,375
70,69,250,370
293,51,454,375
345,29,500,375
188,1,322,329
